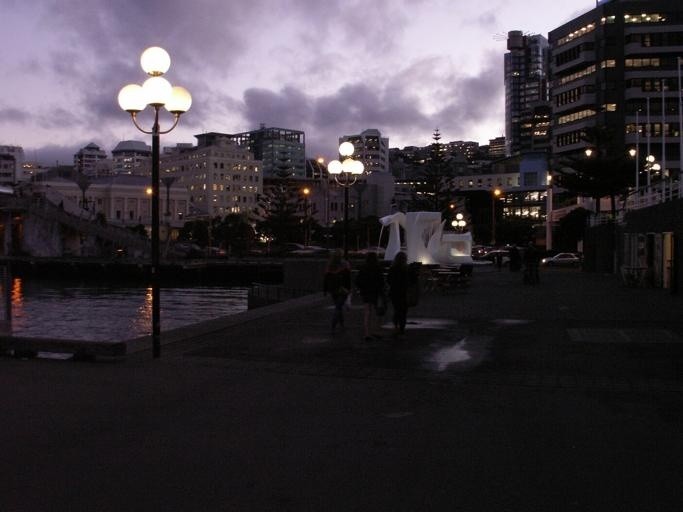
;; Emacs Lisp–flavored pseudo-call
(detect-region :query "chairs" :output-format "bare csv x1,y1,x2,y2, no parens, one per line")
417,263,473,295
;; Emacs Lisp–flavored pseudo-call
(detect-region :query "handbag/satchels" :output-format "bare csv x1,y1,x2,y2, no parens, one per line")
376,307,386,316
336,286,348,295
406,286,417,306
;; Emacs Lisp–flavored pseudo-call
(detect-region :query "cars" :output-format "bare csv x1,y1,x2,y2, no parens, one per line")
162,239,527,268
540,253,583,268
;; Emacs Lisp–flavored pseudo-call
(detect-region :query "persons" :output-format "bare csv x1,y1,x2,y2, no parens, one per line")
490,234,539,286
320,245,351,332
356,250,385,339
387,252,409,334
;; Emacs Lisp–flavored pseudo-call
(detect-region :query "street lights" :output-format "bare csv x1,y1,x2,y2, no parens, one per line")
326,135,362,295
114,45,194,361
303,185,312,248
449,212,467,234
490,190,503,250
584,137,662,218
